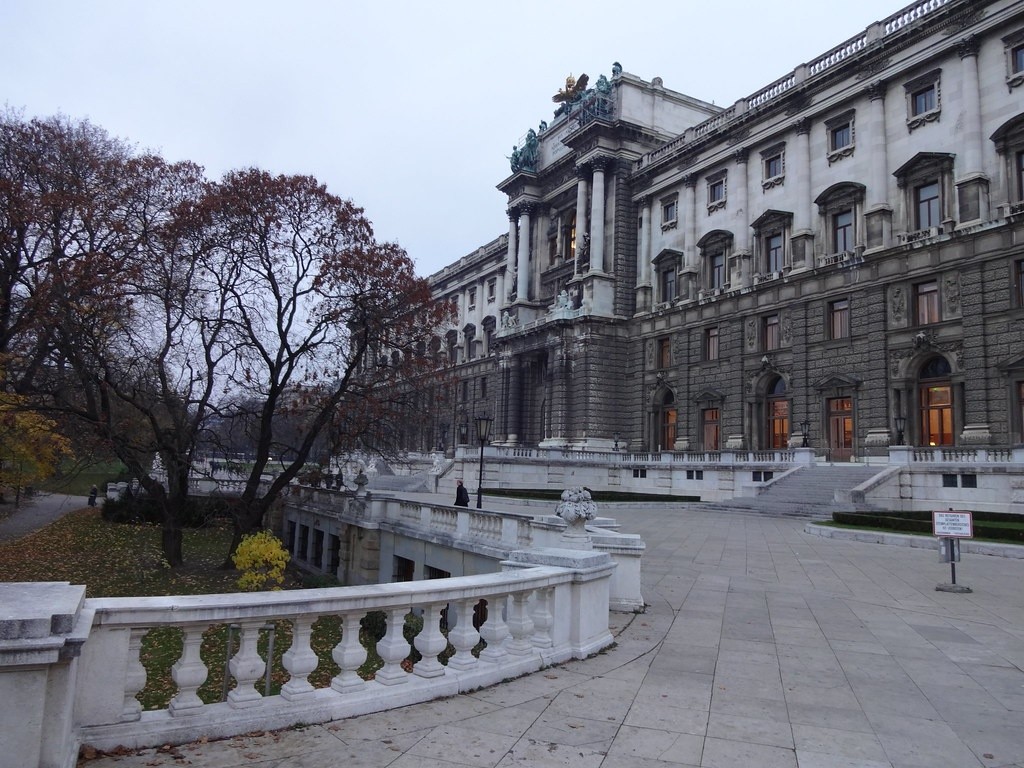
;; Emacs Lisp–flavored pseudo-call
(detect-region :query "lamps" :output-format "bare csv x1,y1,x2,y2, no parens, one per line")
460,424,468,439
800,420,810,436
894,414,906,432
613,431,620,442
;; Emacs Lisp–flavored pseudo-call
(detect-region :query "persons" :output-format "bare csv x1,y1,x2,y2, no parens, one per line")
578,232,590,265
195,456,241,477
298,467,319,488
454,480,470,507
335,471,343,491
325,470,334,490
503,75,613,172
88,484,98,507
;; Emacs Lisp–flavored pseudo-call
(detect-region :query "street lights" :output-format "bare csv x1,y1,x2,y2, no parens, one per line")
475,410,496,510
